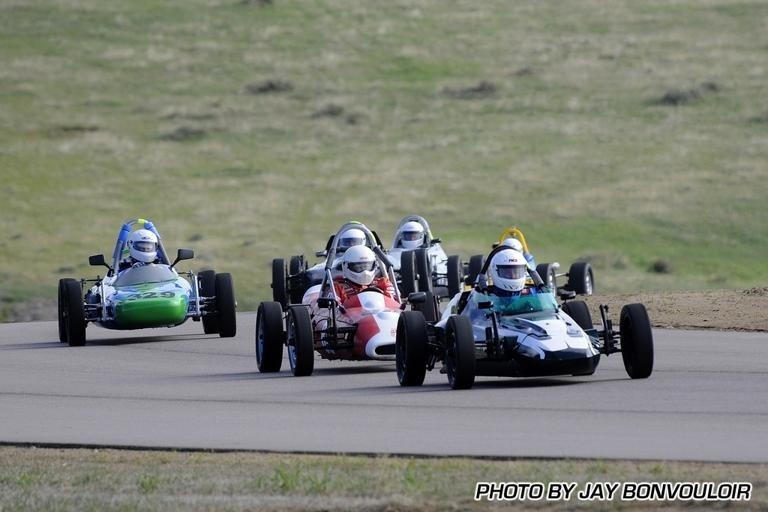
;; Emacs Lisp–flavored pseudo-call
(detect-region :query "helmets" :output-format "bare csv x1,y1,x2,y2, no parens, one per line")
490,239,527,291
401,221,424,248
339,229,378,285
127,230,158,263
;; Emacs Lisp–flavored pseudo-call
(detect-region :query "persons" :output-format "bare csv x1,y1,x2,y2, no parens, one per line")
489,249,543,307
328,245,398,307
500,238,523,255
338,228,366,255
395,222,427,249
119,229,163,268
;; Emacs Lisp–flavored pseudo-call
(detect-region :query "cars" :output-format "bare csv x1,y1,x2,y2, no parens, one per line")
56,218,237,347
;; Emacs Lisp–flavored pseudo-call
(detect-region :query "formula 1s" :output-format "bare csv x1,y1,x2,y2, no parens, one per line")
252,214,654,390
